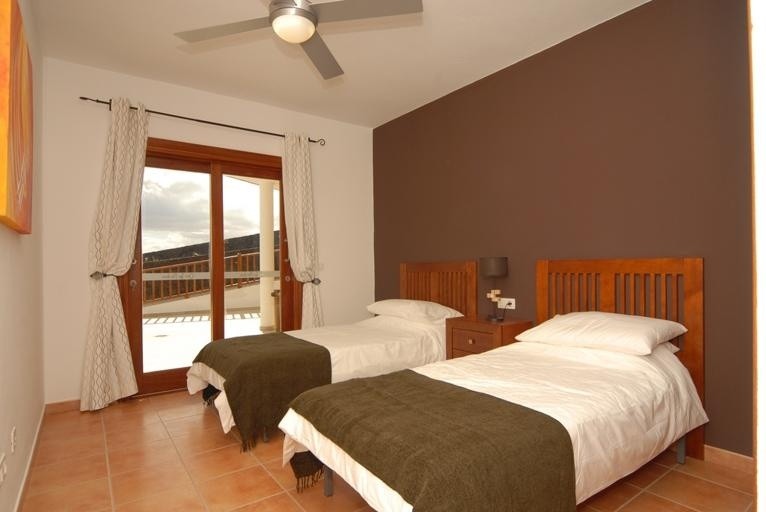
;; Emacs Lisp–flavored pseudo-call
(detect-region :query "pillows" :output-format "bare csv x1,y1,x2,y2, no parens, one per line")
666,343,681,353
367,300,462,323
514,310,688,354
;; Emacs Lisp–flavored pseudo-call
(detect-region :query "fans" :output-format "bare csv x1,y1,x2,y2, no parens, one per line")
174,0,428,80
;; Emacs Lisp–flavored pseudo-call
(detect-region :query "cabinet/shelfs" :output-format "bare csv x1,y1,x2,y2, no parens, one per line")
445,315,532,360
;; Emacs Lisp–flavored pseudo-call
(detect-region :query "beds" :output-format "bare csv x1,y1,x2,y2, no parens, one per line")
279,253,712,512
185,260,480,452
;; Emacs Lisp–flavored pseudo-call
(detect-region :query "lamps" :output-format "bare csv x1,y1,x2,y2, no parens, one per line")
269,8,318,44
479,256,508,320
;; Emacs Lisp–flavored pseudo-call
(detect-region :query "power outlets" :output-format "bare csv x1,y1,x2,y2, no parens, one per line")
498,299,516,309
